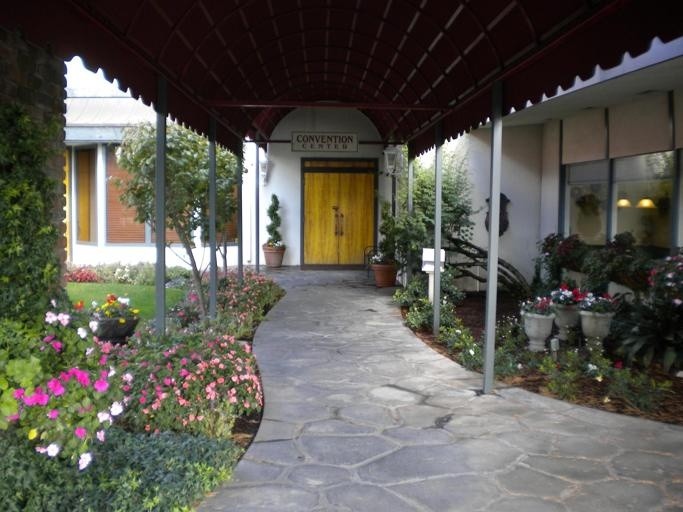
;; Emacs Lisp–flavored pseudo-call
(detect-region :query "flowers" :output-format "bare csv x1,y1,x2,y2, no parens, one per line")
520,230,683,319
88,291,141,327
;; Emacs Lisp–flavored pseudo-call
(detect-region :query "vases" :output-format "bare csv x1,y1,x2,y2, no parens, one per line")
94,317,138,344
513,301,616,356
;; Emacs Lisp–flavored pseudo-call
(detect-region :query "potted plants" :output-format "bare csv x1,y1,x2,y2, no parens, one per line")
262,192,286,269
368,198,400,291
